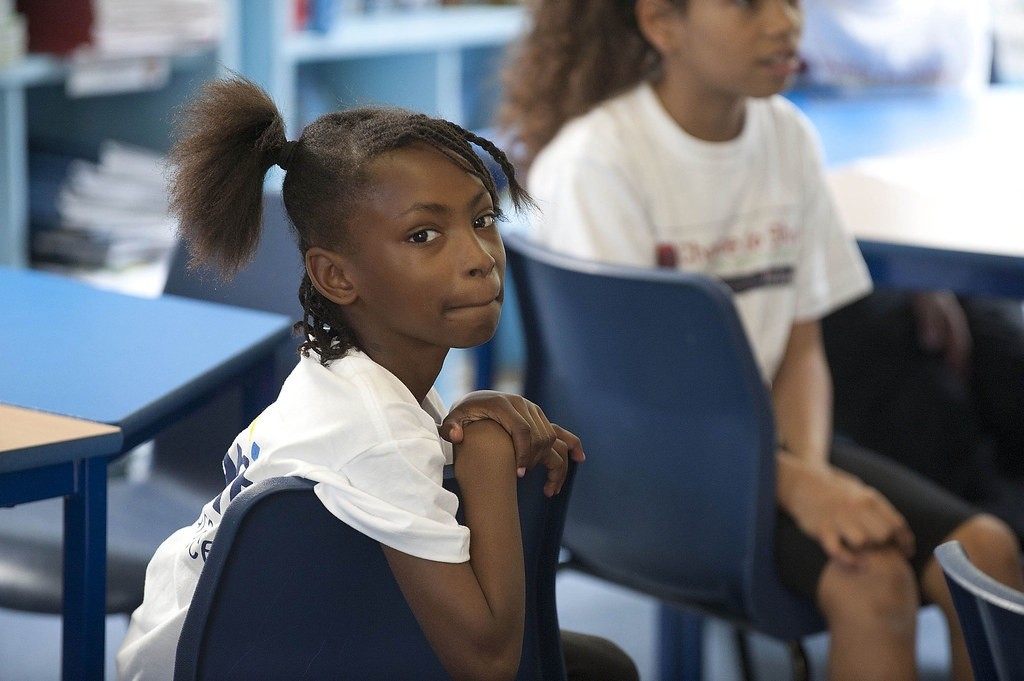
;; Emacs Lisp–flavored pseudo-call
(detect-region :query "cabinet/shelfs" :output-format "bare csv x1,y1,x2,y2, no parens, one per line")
0,0,541,293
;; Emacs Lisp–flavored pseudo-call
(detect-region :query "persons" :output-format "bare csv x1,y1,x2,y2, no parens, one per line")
114,69,586,681
498,0,1023,681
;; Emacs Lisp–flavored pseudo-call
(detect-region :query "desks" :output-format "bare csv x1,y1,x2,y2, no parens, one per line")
0,402,123,681
831,112,1024,300
0,260,292,681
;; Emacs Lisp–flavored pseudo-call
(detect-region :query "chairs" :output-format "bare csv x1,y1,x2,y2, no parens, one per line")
503,228,830,680
174,449,579,681
0,186,312,617
